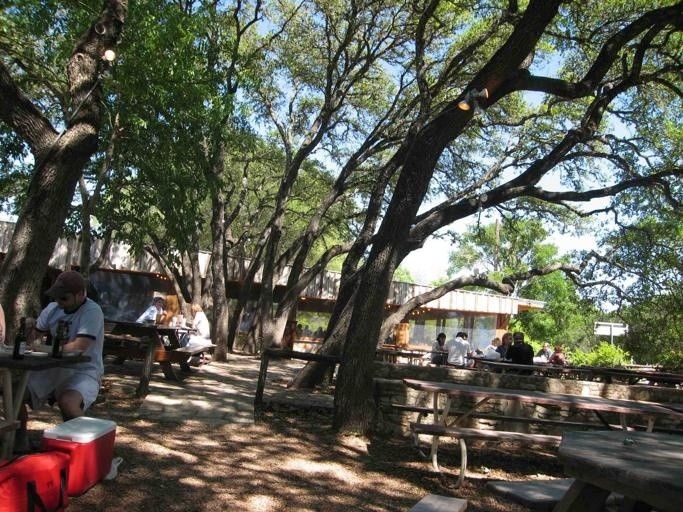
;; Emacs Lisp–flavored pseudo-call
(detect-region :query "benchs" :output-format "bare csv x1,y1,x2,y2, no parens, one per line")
483,476,624,507
393,402,636,438
166,338,218,360
408,423,560,491
408,495,468,512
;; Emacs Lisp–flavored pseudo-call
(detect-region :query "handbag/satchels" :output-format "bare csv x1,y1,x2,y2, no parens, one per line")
0,450,72,512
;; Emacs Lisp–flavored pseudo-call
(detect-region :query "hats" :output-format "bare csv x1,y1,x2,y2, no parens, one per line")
44,270,86,297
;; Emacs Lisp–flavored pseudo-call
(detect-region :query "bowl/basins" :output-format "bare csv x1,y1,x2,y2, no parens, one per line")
145,320,155,323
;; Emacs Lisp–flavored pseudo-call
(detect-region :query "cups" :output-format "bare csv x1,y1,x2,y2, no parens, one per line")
171,317,176,327
180,319,185,327
176,315,183,326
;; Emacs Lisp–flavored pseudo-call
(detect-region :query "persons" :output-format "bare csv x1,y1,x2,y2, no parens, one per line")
295,322,326,337
432,330,565,375
0,302,5,351
28,272,106,419
134,295,210,364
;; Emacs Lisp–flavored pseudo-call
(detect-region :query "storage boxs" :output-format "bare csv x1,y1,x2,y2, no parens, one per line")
41,416,116,500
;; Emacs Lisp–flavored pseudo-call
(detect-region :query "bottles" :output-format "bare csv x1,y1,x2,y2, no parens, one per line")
51,319,65,359
13,317,27,360
